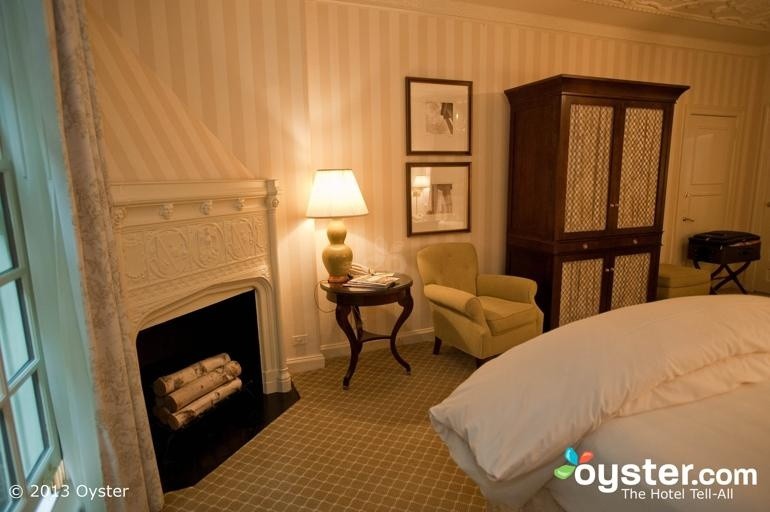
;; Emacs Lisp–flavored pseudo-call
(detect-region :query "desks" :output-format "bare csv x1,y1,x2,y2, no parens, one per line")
317,272,419,389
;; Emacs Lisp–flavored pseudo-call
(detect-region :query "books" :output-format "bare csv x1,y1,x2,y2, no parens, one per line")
343,275,399,289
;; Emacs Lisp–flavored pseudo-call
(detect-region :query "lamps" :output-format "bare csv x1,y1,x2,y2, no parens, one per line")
303,168,371,284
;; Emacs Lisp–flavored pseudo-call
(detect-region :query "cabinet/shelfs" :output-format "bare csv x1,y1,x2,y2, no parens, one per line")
503,71,692,328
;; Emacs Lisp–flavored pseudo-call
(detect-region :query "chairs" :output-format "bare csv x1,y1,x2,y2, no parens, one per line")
414,241,546,367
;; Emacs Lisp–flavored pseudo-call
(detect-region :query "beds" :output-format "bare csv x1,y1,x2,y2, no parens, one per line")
546,380,770,511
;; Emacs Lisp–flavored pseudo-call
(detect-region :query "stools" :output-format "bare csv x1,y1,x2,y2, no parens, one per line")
688,229,762,295
657,261,710,301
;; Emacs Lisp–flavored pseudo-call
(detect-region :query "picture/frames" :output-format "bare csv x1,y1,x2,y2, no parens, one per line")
404,75,473,157
406,161,473,235
